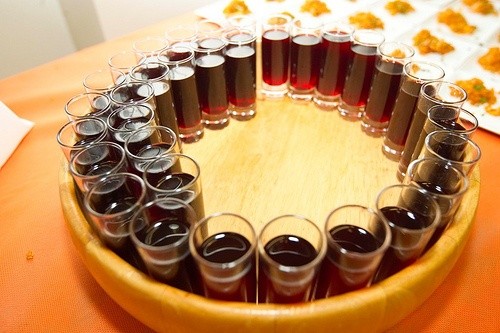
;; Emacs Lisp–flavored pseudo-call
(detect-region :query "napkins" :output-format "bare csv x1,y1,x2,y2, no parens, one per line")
0,98,33,170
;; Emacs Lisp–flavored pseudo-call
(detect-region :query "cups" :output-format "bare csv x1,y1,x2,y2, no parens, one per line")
55,16,480,298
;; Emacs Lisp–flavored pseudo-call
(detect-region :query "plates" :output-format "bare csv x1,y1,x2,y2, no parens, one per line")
57,95,481,333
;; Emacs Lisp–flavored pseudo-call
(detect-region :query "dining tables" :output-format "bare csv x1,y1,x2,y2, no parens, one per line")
0,15,500,333
